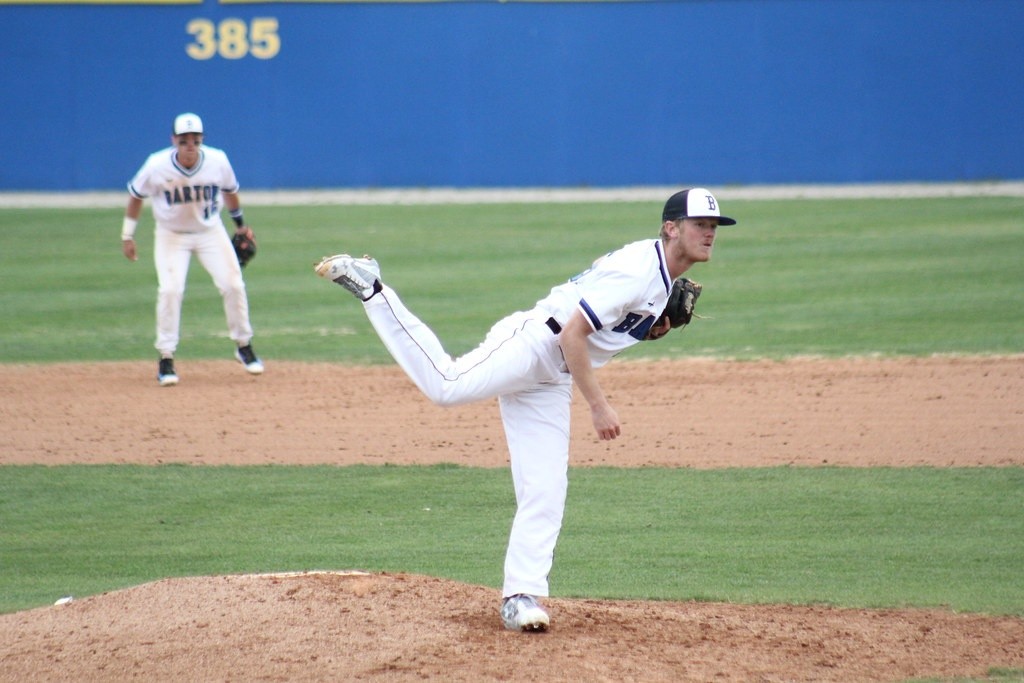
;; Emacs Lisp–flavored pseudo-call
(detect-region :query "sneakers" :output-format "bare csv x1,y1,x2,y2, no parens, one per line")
157,358,179,385
234,342,262,373
316,254,382,298
500,594,550,633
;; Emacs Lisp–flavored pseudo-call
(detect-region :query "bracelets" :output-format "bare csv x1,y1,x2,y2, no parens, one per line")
122,236,132,241
122,218,137,235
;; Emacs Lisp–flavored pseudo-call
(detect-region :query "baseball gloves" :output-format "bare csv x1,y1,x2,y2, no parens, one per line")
655,276,700,327
230,229,257,268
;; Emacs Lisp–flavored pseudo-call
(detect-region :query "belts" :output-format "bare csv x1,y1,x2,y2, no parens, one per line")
545,317,569,373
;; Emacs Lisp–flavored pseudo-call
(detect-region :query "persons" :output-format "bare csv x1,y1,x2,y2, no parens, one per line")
121,113,263,386
315,187,735,631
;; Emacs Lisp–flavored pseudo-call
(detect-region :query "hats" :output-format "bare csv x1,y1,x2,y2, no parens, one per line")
174,113,202,135
662,188,736,226
